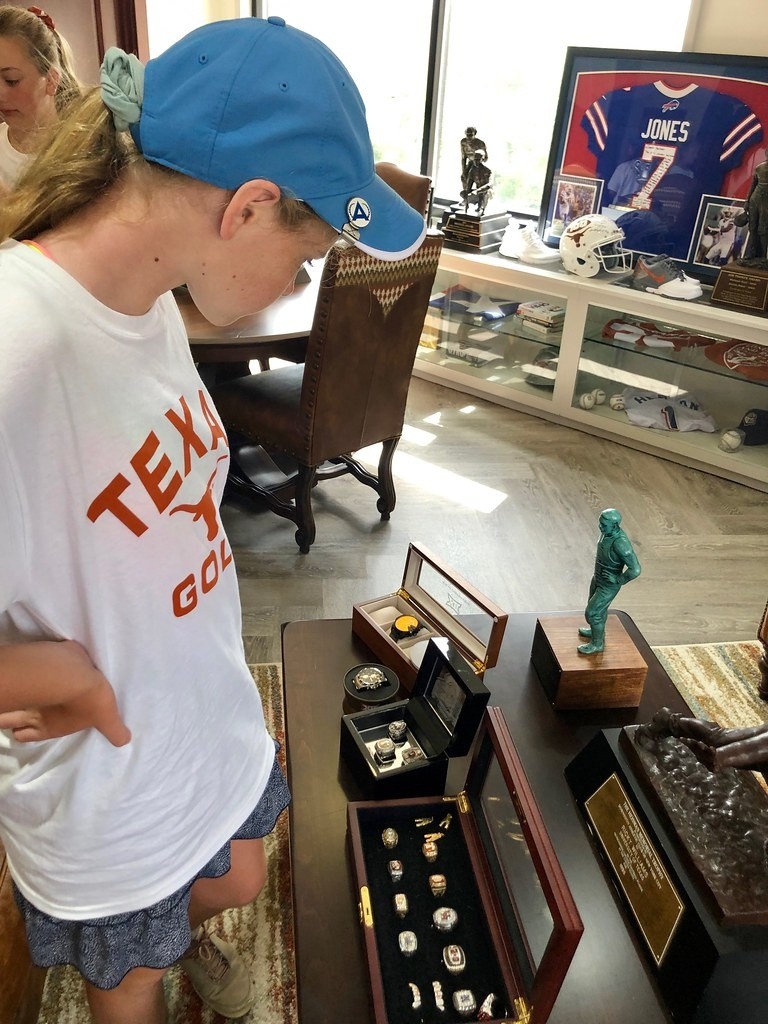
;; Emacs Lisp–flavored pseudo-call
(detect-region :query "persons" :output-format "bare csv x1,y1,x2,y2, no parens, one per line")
577,509,641,654
460,127,492,212
0,15,427,1024
697,208,744,265
639,707,767,772
0,5,82,192
734,149,768,260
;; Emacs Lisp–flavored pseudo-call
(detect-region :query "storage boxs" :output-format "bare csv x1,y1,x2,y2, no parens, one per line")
349,540,508,700
344,704,585,1023
340,637,491,800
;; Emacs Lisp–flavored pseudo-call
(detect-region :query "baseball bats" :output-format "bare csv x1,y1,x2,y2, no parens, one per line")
467,183,494,196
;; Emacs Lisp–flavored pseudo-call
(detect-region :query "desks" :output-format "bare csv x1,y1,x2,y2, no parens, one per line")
171,240,328,513
282,609,768,1024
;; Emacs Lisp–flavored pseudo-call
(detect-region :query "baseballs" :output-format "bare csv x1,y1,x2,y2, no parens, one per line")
579,392,595,410
722,431,741,450
609,394,626,410
591,388,606,405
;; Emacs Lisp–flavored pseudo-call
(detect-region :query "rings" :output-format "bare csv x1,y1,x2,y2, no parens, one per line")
395,893,409,918
375,737,396,756
388,720,407,737
433,907,458,932
401,746,424,764
388,860,403,881
398,931,508,1022
382,828,398,848
422,842,438,862
429,875,447,896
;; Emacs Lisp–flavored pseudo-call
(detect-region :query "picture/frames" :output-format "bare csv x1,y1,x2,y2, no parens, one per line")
537,46,768,286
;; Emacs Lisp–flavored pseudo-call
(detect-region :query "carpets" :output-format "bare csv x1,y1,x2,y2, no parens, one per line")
649,639,768,795
37,665,297,1024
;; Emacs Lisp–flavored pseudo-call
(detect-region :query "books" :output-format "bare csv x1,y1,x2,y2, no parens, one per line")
514,300,566,334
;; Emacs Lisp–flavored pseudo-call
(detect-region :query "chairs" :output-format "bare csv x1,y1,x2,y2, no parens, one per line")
209,230,445,553
375,162,434,224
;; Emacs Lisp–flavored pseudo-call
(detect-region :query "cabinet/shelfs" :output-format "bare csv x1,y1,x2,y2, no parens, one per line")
412,247,768,496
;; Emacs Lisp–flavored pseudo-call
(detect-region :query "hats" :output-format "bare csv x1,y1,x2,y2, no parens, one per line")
732,408,768,446
128,16,427,261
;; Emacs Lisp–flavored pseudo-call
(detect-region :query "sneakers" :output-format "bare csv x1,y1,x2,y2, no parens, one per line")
177,921,255,1016
631,252,703,300
633,253,700,287
499,218,561,264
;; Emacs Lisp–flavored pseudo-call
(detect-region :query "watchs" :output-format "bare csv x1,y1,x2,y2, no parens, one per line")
389,614,424,643
352,667,388,690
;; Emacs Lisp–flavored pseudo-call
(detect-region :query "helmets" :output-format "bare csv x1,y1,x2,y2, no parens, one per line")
526,347,560,385
615,209,669,267
559,214,626,277
721,207,734,220
